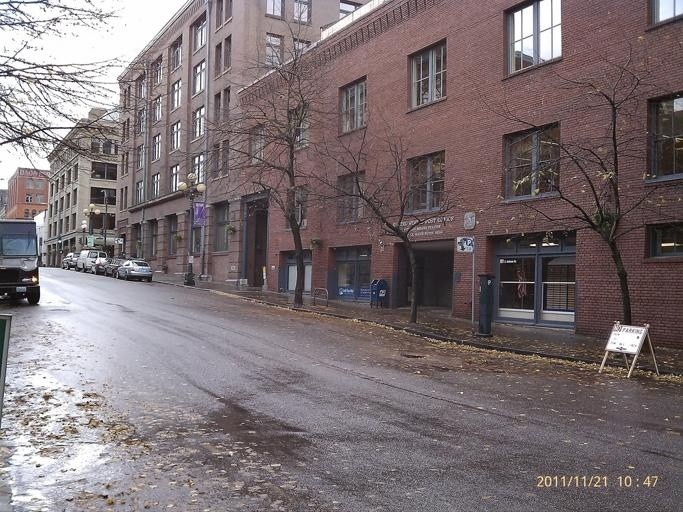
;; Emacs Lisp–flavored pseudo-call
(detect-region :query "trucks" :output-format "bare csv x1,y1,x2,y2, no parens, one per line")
0,219,40,304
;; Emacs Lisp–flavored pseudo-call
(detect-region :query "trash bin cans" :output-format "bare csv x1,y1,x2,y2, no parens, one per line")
371,280,388,309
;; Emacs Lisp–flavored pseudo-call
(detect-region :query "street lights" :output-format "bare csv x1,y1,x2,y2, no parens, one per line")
178,172,207,285
81,203,100,247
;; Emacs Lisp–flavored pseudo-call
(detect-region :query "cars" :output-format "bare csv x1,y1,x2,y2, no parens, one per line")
62,248,152,282
